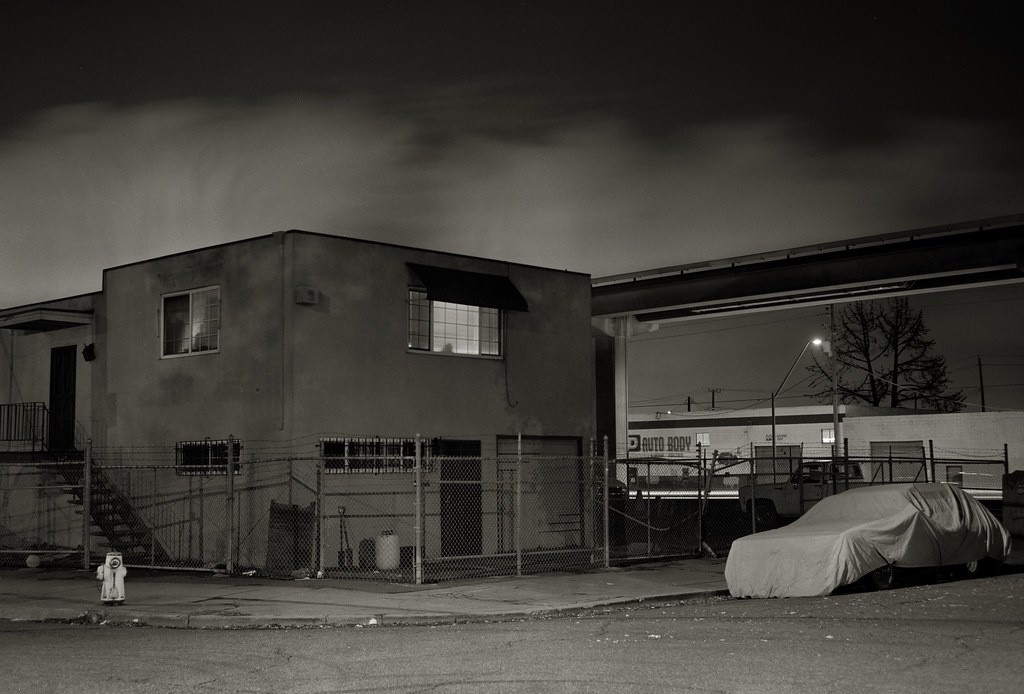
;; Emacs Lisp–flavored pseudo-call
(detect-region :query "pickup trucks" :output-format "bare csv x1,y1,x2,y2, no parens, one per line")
737,458,882,531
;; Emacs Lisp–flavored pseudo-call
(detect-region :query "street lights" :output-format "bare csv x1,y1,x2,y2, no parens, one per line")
771,337,822,485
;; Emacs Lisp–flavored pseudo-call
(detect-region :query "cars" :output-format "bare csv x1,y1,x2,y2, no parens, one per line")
724,480,1012,599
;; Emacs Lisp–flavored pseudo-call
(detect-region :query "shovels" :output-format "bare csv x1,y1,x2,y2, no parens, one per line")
337,505,353,568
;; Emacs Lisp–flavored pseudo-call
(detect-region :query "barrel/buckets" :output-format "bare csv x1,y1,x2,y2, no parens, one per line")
359,531,416,569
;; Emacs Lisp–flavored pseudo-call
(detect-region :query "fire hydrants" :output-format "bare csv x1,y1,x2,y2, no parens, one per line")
94,551,129,606
